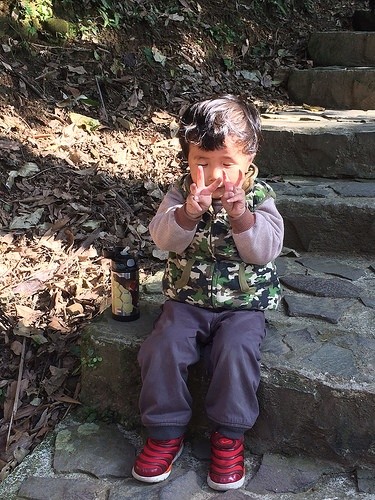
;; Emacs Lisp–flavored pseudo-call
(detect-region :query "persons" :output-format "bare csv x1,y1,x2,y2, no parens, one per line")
128,94,291,493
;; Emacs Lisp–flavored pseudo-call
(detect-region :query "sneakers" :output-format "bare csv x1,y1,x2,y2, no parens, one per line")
130,431,187,483
204,428,247,492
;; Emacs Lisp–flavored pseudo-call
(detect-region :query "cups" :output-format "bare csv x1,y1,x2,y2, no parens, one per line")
103,246,140,322
